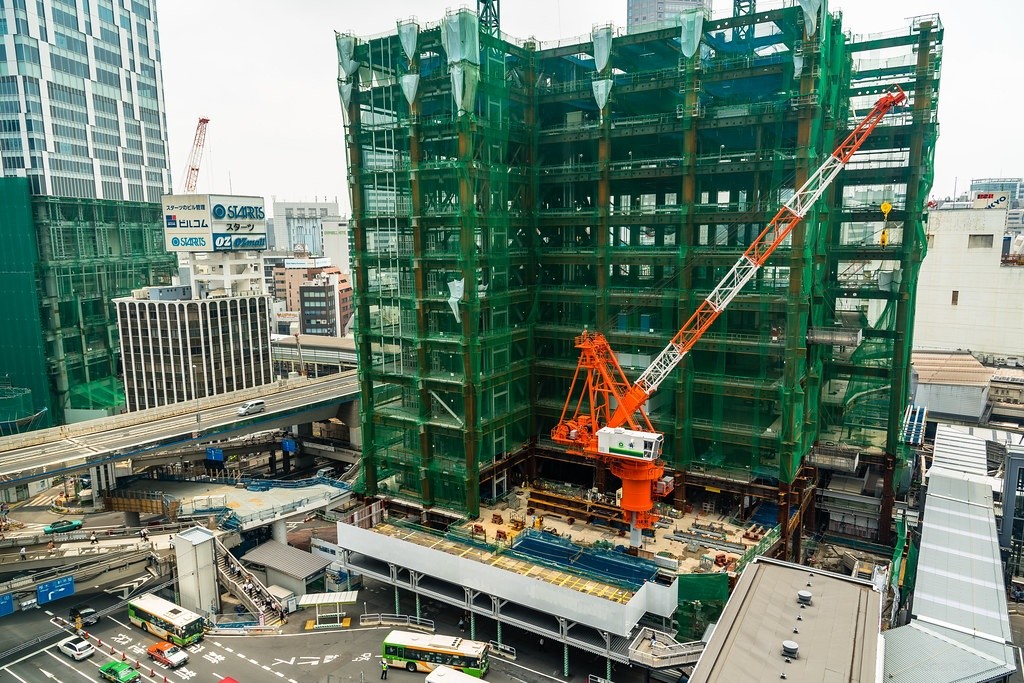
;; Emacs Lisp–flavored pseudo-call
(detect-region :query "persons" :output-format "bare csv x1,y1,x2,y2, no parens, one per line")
532,516,535,529
458,616,465,632
47,540,55,554
139,527,150,542
90,531,98,544
20,547,26,560
223,553,285,624
379,661,390,680
169,535,174,549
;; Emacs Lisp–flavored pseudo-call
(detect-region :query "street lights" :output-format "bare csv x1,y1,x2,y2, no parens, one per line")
193,365,200,431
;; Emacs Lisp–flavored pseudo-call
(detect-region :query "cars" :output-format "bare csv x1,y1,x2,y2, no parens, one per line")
69,605,100,627
98,659,141,683
147,641,189,669
44,519,83,534
56,635,96,661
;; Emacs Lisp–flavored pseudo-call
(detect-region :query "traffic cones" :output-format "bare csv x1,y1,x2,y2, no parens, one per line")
110,647,115,654
150,669,155,677
135,660,141,669
122,653,126,661
98,639,103,647
163,676,168,683
84,632,89,639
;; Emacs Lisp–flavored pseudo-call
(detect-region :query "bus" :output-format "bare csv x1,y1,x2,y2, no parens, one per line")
424,665,490,683
128,592,206,648
382,630,491,679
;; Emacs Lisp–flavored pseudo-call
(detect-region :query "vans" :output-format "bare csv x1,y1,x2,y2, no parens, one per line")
238,400,266,415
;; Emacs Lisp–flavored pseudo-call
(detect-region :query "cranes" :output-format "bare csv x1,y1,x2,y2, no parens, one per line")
551,81,908,481
